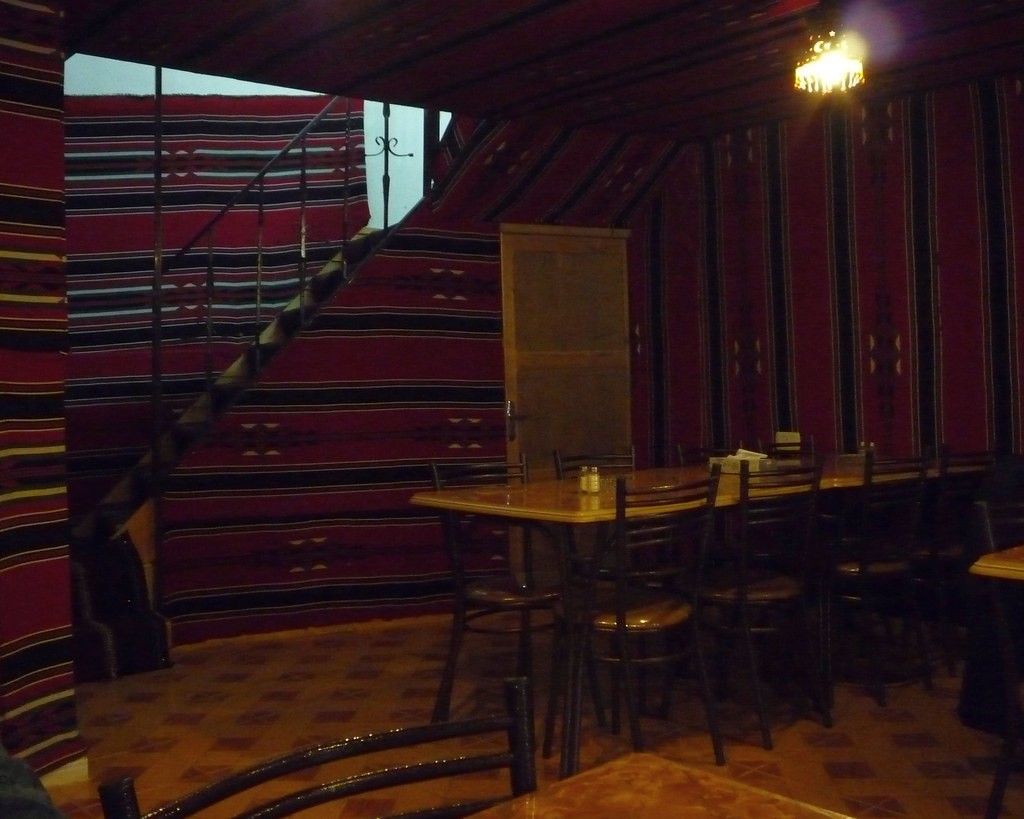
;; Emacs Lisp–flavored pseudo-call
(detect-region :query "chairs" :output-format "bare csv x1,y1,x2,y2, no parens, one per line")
431,436,1003,764
97,675,538,819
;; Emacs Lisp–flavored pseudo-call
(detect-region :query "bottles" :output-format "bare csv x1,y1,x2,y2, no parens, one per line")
857,442,866,453
579,465,600,494
868,442,875,447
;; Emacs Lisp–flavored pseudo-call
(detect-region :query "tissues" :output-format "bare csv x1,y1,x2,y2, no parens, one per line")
708,448,779,474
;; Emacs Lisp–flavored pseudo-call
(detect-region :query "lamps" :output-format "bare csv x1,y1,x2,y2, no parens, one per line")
795,22,865,95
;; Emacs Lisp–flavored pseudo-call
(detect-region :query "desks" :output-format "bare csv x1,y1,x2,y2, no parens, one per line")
459,752,853,819
970,544,1024,818
408,451,989,781
